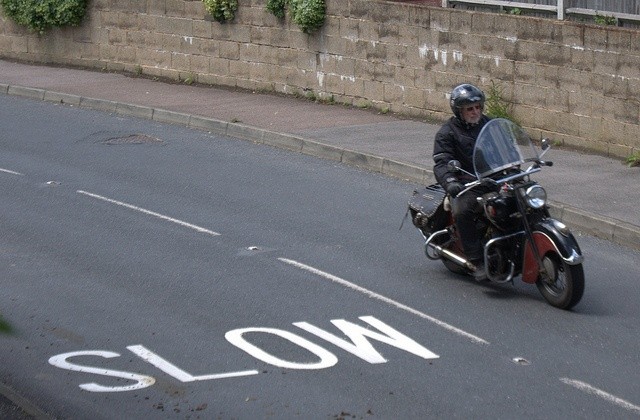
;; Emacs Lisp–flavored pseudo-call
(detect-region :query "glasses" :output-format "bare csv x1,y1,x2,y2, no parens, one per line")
463,106,481,111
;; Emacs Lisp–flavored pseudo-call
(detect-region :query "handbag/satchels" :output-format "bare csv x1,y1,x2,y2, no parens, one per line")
408,187,447,244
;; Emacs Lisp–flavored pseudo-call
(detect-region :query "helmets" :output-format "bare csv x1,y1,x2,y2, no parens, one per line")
449,83,484,116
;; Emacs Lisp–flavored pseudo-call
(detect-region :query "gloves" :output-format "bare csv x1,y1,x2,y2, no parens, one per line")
444,181,466,198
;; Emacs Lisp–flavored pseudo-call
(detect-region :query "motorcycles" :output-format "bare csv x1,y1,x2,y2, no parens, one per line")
406,116,586,310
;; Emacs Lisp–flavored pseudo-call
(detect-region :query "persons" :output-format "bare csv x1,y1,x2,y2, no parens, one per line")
432,83,524,282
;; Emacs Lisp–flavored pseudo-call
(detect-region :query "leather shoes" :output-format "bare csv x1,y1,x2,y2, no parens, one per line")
474,255,497,282
